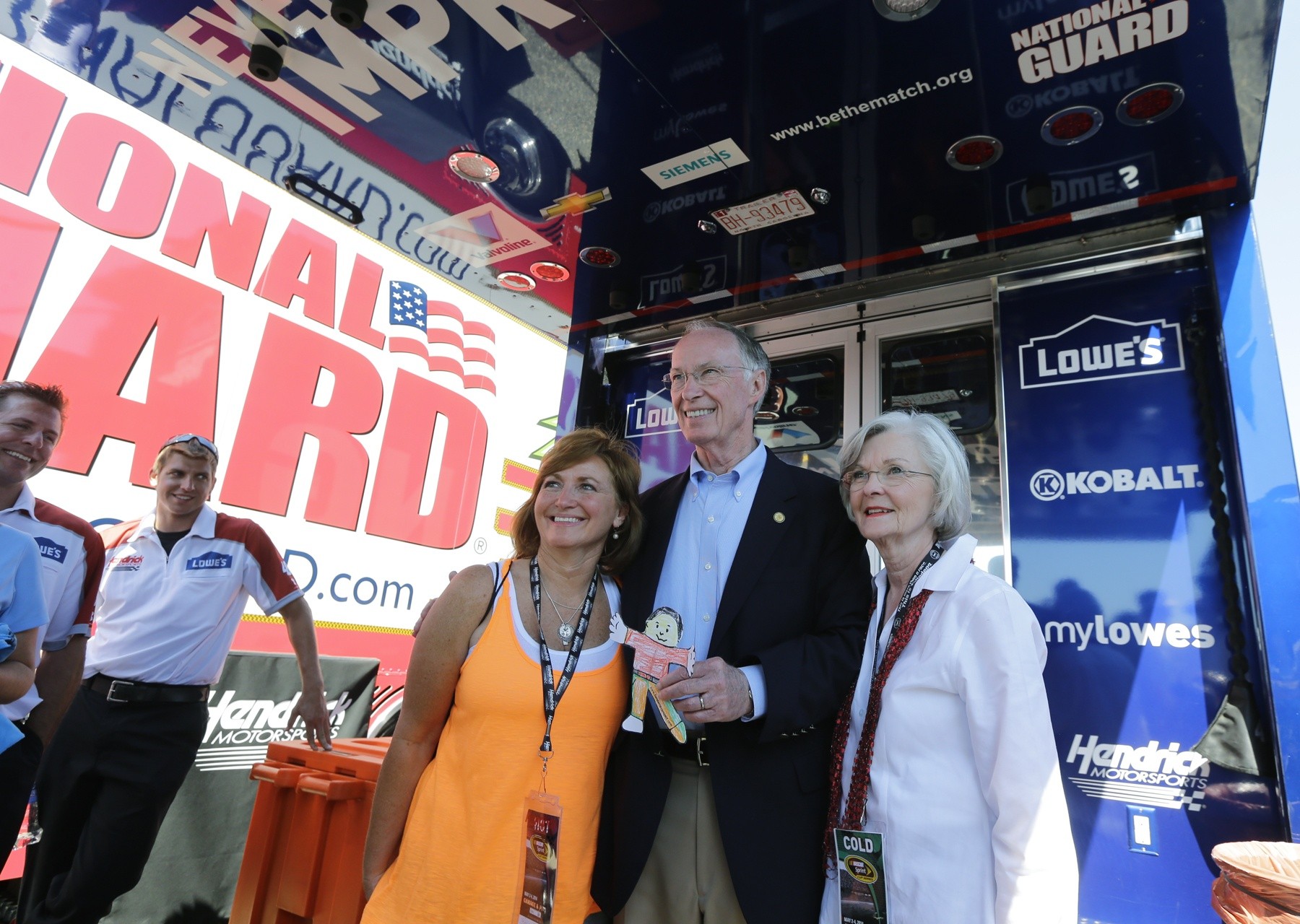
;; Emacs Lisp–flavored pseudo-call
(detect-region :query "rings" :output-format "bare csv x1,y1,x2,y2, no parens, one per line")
699,695,705,711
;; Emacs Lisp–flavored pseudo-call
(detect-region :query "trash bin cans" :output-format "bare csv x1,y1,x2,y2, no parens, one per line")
1208,840,1300,924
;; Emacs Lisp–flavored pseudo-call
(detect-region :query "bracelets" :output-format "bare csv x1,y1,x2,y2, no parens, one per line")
745,687,755,718
408,318,882,924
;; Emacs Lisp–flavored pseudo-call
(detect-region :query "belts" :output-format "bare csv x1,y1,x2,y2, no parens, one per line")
80,673,210,704
662,735,709,766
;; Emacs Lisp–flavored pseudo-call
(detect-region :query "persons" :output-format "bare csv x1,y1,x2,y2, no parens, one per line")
0,523,51,755
0,380,107,884
16,432,334,924
815,410,1082,924
358,428,646,923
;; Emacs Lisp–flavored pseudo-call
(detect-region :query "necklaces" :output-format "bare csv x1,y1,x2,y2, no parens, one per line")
538,571,590,646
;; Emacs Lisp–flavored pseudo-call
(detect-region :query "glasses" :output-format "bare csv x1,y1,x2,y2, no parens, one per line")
662,364,754,391
158,433,219,464
841,465,938,492
